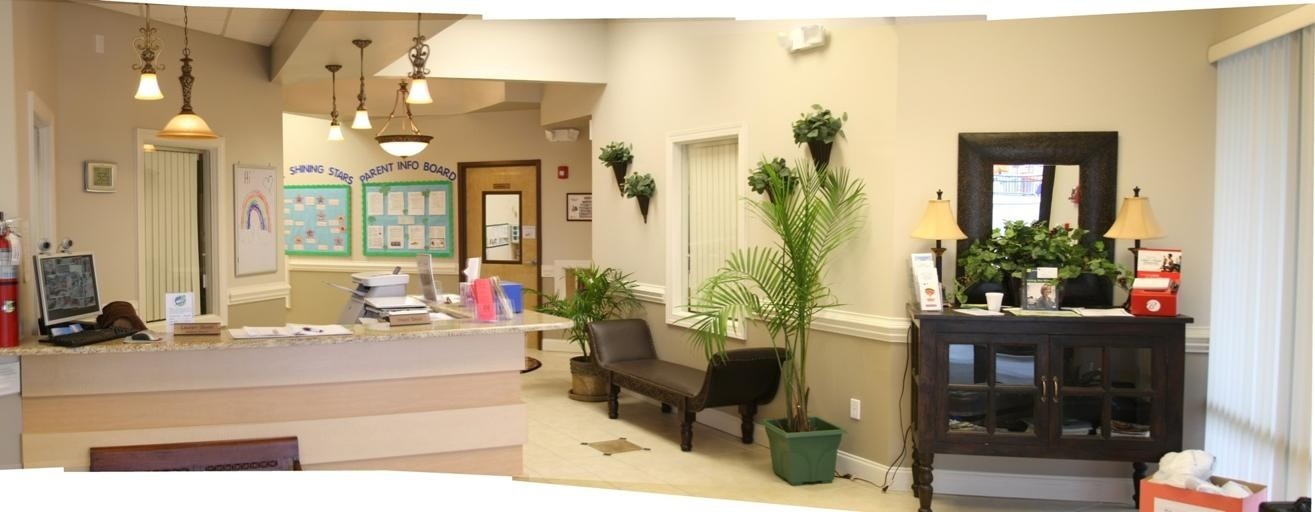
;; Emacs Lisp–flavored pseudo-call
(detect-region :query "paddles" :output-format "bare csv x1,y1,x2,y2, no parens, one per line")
519,357,542,373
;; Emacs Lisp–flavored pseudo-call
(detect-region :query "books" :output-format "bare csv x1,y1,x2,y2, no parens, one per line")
496,281,521,314
1139,474,1267,512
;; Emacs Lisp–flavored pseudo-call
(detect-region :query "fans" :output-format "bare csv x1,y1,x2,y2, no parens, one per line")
233,165,277,276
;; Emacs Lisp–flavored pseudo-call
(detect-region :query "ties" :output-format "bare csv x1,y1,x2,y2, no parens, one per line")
32,252,104,337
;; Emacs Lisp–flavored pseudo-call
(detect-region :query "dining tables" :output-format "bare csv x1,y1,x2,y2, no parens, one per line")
671,153,870,486
946,220,1135,305
519,262,646,402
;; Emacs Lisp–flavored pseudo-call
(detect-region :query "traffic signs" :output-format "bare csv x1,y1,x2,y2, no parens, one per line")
850,398,860,420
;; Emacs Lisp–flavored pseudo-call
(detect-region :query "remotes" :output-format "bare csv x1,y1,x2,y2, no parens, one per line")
133,330,161,342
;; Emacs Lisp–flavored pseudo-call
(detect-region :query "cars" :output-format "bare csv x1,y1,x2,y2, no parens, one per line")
985,292,1005,312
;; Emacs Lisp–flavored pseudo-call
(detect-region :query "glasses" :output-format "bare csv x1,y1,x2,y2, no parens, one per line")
1103,186,1168,310
374,79,434,161
156,6,218,139
132,4,164,100
405,13,433,104
912,189,968,308
143,144,155,153
351,40,372,129
326,65,342,141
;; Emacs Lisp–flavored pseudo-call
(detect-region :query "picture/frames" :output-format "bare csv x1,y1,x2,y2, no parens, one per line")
907,306,1194,511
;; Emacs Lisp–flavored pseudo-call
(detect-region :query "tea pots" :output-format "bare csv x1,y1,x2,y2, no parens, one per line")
958,132,1117,309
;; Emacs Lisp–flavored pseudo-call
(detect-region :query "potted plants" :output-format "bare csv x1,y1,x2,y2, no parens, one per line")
1021,267,1059,311
85,160,118,193
567,193,592,221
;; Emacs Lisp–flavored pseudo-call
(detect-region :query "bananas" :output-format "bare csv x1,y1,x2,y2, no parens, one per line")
53,326,139,347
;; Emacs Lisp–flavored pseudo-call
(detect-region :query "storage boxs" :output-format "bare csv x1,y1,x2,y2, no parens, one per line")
584,319,791,451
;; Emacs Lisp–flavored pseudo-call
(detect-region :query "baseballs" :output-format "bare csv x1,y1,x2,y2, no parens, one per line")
0,213,23,348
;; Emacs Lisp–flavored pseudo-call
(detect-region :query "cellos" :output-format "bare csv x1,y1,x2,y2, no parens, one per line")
322,265,410,324
